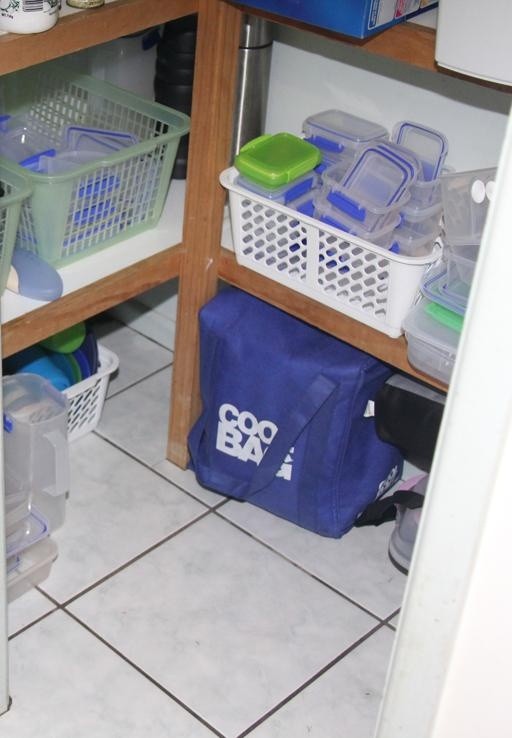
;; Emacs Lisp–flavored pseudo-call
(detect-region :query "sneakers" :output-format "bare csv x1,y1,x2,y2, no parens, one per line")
386,475,433,577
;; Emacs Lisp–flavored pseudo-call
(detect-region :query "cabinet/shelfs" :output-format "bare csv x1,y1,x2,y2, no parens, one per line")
0,0,512,470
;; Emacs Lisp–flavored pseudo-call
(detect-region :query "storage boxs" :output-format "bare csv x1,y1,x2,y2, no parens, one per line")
3,372,73,605
234,109,444,310
225,0,441,42
401,166,499,382
0,111,143,244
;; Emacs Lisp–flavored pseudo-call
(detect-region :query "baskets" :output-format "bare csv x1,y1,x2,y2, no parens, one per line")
0,175,33,297
219,141,448,338
0,65,190,271
0,61,193,296
7,338,120,447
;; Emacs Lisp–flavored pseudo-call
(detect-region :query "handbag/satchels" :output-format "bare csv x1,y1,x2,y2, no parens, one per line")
186,287,405,540
